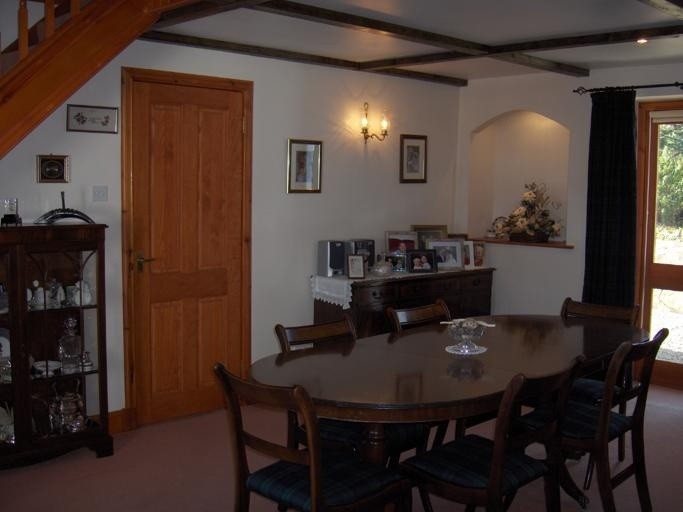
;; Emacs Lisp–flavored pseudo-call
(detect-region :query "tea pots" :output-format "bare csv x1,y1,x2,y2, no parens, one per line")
65,415,95,434
371,252,395,278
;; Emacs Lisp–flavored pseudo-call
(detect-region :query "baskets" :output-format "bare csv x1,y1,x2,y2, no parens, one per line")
507,228,551,244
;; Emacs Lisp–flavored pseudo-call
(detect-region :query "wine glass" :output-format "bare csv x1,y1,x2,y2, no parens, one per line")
444,358,486,383
442,319,488,355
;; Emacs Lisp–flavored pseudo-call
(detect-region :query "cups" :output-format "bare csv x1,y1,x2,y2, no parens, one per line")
4,197,18,214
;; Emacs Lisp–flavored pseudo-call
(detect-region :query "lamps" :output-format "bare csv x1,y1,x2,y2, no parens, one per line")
361,102,388,143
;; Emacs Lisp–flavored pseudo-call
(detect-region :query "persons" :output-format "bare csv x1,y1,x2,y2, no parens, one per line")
386,258,392,268
444,249,455,263
419,256,430,269
413,257,421,269
392,242,407,254
394,259,402,267
436,250,446,263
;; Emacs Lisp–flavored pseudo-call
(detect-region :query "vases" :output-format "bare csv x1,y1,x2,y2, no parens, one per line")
509,232,549,243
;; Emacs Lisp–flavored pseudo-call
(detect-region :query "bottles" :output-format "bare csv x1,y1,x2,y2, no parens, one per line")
0,280,96,383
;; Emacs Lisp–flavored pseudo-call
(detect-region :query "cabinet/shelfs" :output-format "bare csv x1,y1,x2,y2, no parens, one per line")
312,265,496,348
0,222,115,470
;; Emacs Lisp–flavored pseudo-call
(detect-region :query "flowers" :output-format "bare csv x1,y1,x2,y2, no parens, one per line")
492,182,561,241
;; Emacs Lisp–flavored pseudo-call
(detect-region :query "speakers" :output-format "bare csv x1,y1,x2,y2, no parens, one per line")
318,240,344,277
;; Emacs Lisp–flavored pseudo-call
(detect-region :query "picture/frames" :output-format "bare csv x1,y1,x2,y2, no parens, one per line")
348,223,486,280
286,137,323,193
66,103,118,133
400,133,428,183
34,154,70,183
